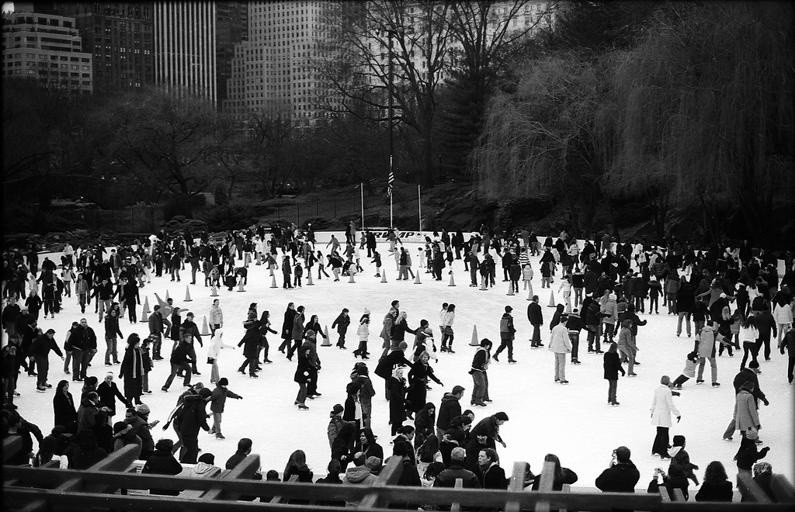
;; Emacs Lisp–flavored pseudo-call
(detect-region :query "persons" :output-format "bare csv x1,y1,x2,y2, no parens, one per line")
0,218,794,511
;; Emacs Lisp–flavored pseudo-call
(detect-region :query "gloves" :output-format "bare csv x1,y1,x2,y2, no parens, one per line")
239,396,242,399
764,399,769,406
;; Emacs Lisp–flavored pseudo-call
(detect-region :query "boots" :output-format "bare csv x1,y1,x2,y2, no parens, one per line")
41,381,52,388
36,381,46,390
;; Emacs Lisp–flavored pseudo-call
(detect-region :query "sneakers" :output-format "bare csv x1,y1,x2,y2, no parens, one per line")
492,354,498,361
239,342,293,377
697,380,704,382
13,391,20,396
441,346,455,353
18,367,38,375
217,433,224,438
336,344,370,359
508,359,517,362
105,362,112,366
555,379,569,383
571,339,614,366
295,392,322,410
126,372,201,408
471,398,492,407
712,382,720,386
65,370,87,381
114,360,120,364
623,358,640,376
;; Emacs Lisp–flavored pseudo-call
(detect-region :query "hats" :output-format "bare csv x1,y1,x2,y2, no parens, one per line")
746,426,758,440
425,328,432,334
452,448,464,461
573,309,579,314
220,378,228,385
137,404,150,415
421,320,428,326
334,404,343,414
104,372,113,378
399,341,407,348
364,308,370,314
673,436,685,446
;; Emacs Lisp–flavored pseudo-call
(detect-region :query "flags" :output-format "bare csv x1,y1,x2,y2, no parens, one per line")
386,170,395,198
